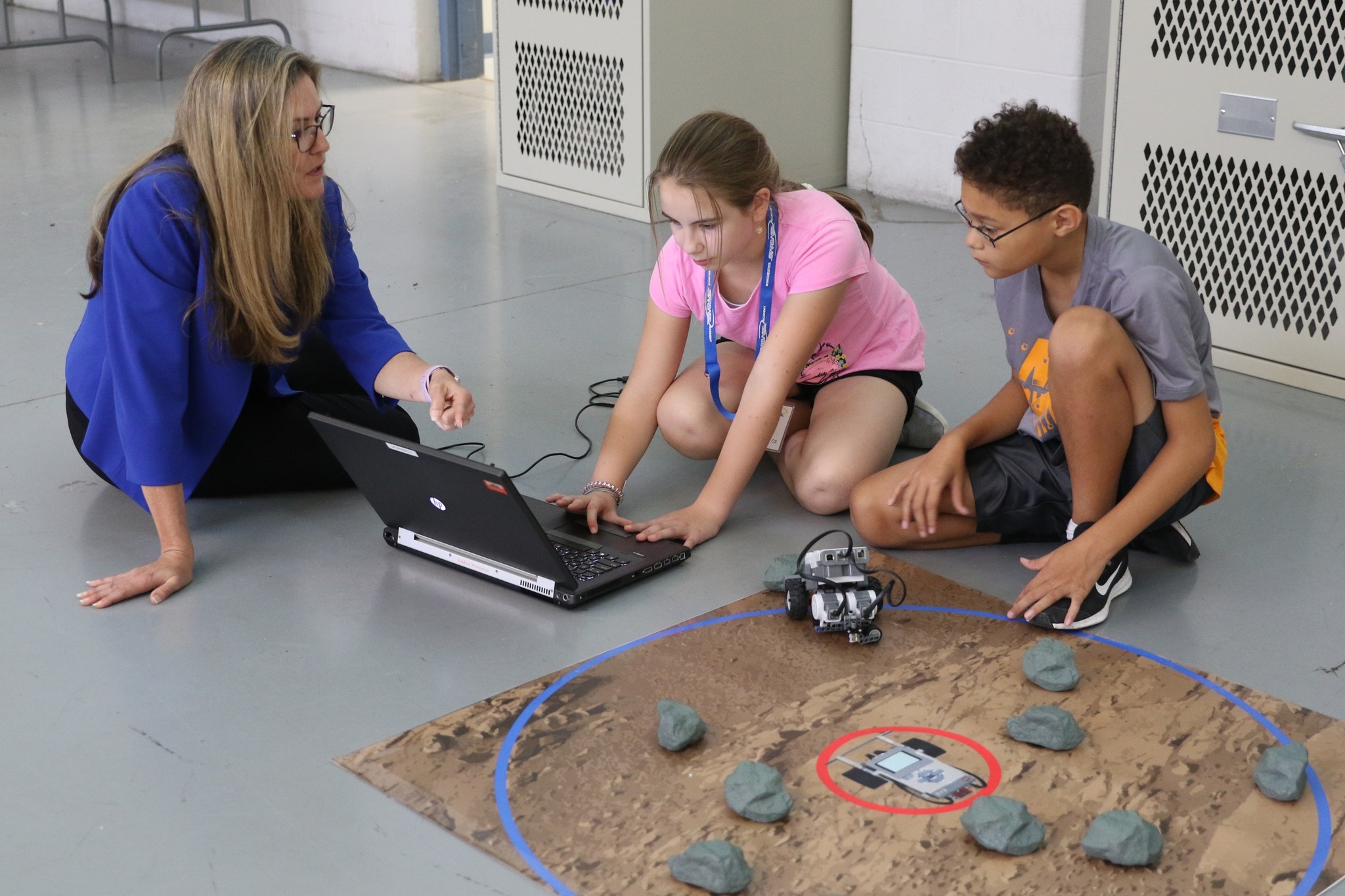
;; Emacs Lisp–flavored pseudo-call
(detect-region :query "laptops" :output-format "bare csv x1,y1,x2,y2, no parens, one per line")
302,411,691,607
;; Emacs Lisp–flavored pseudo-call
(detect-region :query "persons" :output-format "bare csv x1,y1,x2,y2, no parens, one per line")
65,35,475,608
546,110,947,550
849,99,1229,629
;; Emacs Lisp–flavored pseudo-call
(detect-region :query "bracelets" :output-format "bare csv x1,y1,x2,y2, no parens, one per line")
420,365,461,404
582,482,624,507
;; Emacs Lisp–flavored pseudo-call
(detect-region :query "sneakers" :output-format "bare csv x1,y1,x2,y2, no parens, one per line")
897,396,948,450
1026,522,1133,630
1128,520,1201,563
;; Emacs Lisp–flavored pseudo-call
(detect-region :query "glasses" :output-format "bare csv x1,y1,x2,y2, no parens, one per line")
954,199,1061,249
291,104,335,153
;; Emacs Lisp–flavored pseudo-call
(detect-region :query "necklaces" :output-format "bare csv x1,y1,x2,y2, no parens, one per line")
720,292,746,306
